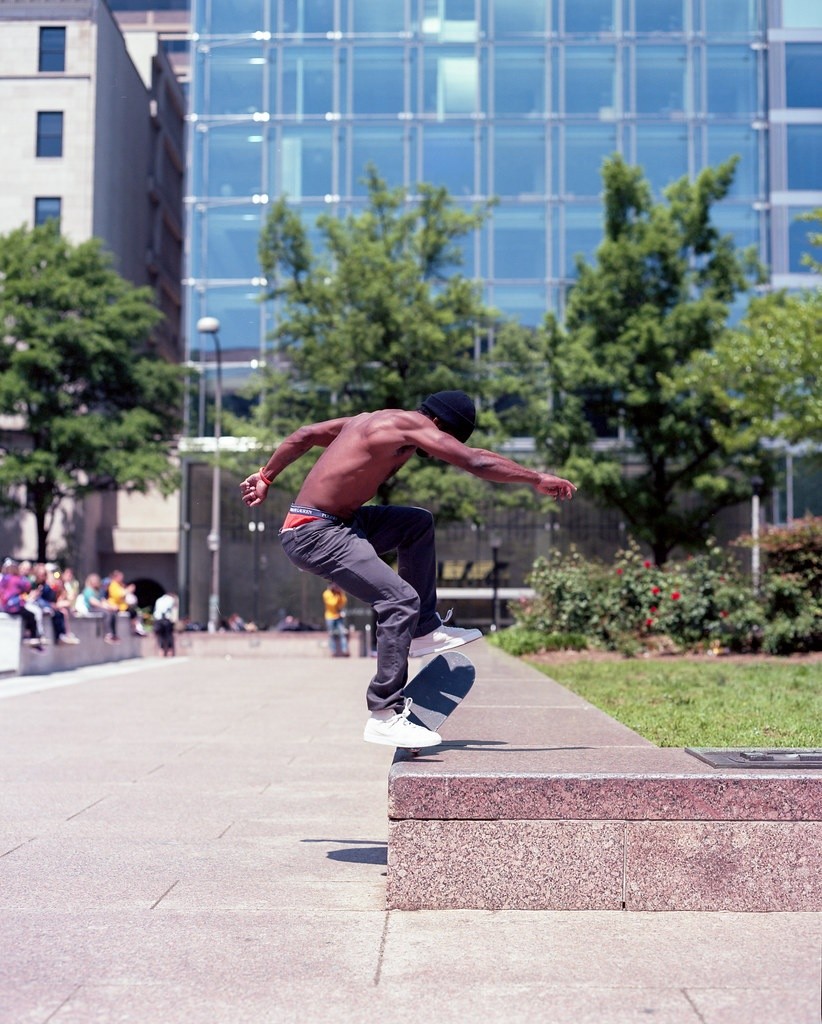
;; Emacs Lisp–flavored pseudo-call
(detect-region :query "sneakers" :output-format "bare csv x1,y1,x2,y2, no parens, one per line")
409,609,482,658
362,697,443,749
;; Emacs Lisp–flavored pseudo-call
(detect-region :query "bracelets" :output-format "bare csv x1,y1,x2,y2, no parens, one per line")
259,466,275,485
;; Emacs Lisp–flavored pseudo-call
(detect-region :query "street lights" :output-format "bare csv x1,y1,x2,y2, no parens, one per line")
195,315,224,632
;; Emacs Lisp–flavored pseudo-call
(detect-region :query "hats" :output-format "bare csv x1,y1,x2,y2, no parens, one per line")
422,388,476,442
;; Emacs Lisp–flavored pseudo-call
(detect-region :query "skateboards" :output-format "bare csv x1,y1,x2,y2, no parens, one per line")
379,648,478,759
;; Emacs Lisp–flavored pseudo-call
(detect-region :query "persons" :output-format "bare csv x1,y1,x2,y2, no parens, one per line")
154,593,178,656
322,579,351,658
0,559,148,655
237,388,577,749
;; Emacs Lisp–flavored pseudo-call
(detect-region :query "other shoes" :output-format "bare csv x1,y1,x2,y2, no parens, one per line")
59,631,79,645
